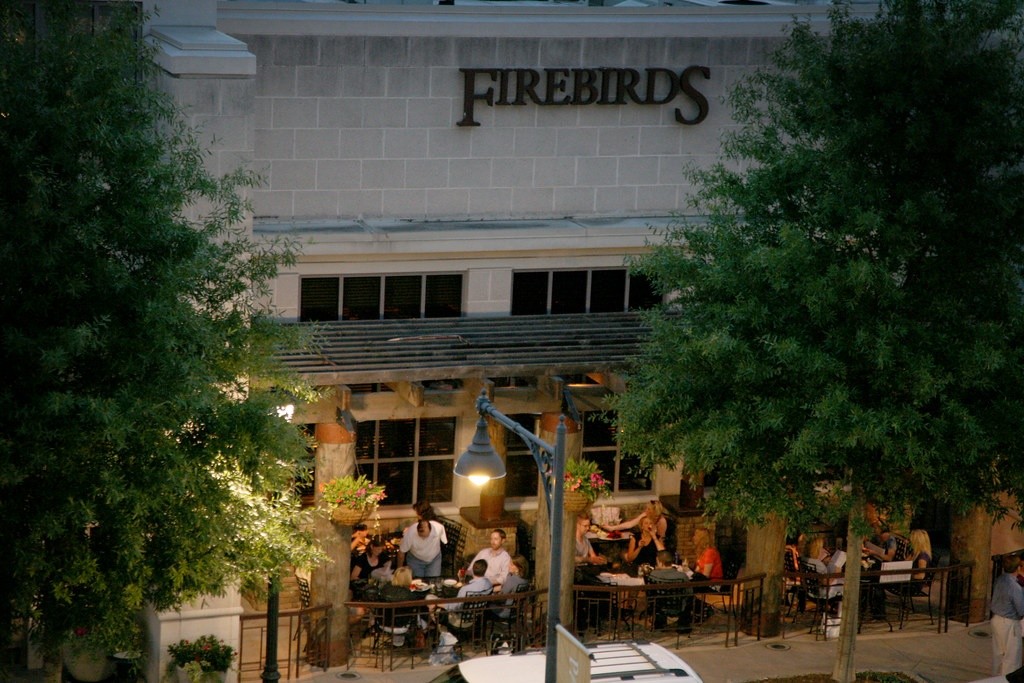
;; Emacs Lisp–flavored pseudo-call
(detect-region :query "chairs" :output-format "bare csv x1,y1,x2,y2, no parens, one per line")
898,546,944,627
783,544,801,616
289,572,311,642
644,570,694,651
370,591,424,656
792,558,843,634
583,574,637,632
878,527,912,613
440,583,494,659
437,513,462,575
704,550,743,619
371,594,421,672
488,582,535,650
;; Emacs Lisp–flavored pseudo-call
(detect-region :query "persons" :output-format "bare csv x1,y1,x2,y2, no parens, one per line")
990,555,1024,676
859,502,931,620
786,533,845,626
574,500,723,643
348,499,529,644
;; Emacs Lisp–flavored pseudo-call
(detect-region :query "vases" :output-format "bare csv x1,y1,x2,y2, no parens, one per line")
112,651,142,683
174,663,226,683
331,503,375,526
562,488,595,513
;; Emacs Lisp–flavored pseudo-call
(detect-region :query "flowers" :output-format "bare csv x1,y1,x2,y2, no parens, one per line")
314,468,390,508
163,636,240,683
549,453,608,500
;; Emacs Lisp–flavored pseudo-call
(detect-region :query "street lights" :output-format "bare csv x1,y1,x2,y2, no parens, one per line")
452,388,568,682
258,391,296,683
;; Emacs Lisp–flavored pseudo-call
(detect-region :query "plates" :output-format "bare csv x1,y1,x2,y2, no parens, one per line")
443,579,457,587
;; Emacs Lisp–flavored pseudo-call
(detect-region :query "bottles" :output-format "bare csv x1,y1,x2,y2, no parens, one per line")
458,570,465,584
675,552,680,564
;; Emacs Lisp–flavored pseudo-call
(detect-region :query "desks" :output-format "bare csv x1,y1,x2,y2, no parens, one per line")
353,534,401,575
844,549,895,635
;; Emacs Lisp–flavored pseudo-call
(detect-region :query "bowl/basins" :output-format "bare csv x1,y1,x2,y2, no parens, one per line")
415,583,428,589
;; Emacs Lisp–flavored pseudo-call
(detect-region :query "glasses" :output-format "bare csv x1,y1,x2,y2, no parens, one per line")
580,523,591,528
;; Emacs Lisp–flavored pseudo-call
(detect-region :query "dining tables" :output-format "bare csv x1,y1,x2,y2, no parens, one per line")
587,527,638,572
404,570,464,636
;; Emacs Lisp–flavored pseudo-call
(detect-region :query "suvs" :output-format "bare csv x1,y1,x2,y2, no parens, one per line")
426,638,704,683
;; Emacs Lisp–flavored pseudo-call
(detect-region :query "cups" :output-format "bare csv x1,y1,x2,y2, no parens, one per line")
682,563,688,572
644,564,650,570
429,577,444,594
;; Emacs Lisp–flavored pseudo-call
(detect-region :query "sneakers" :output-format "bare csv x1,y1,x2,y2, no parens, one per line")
438,632,458,653
